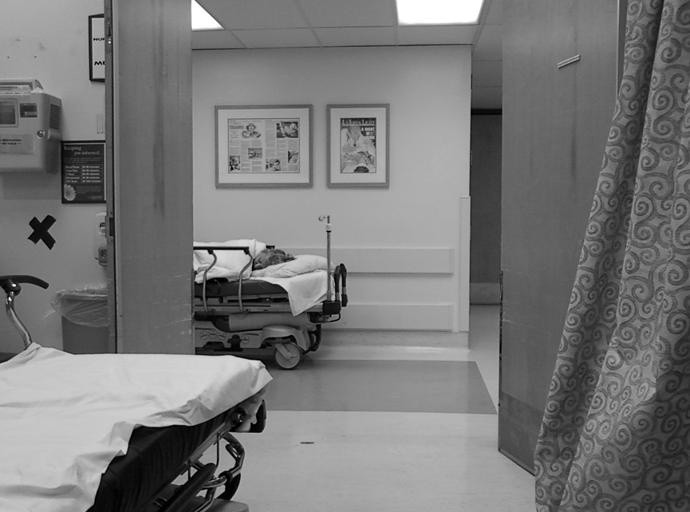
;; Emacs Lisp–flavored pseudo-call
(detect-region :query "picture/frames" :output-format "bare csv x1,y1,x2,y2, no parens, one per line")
88,13,107,82
61,141,107,204
214,104,390,188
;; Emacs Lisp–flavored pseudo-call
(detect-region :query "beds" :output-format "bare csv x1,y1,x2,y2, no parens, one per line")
190,256,347,371
1,341,272,510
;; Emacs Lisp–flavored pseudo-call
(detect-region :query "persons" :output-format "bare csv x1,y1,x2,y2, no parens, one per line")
341,125,353,148
242,123,261,138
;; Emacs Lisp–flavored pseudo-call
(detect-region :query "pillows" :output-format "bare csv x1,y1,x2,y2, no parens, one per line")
191,239,264,277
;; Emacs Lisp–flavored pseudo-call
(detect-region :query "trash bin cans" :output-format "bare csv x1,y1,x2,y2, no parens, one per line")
57,288,108,354
